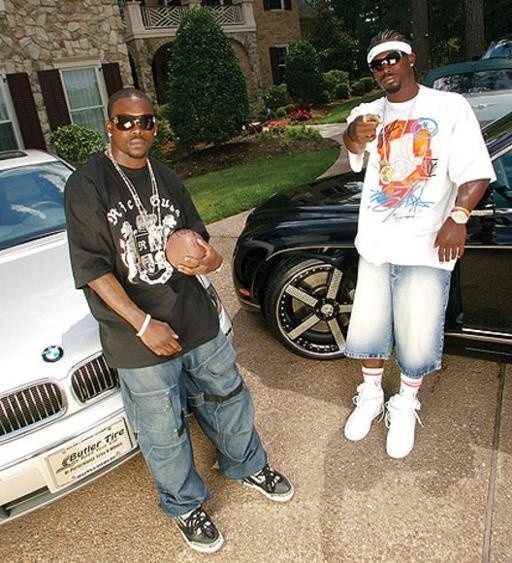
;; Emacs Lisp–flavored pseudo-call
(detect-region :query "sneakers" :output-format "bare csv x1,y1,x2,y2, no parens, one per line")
172,504,224,554
343,379,424,459
239,464,294,504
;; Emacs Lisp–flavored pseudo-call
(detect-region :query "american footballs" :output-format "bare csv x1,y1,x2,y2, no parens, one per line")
168,229,205,270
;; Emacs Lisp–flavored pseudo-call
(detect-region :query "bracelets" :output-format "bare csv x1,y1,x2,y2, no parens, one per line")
213,256,225,272
449,210,469,225
136,312,152,338
449,206,470,216
347,121,360,145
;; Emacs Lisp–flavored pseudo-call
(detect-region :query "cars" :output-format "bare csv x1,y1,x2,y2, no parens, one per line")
0,148,234,525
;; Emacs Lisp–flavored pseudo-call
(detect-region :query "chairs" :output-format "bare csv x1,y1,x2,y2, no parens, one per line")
4,178,54,222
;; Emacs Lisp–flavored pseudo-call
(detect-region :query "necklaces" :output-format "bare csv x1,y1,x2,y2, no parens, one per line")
381,92,416,163
107,145,165,264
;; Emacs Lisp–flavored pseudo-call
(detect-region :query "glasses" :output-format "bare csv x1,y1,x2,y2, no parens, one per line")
109,114,155,130
370,50,408,72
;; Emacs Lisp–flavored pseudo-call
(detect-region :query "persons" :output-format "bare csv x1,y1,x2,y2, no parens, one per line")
342,27,496,460
65,87,294,555
